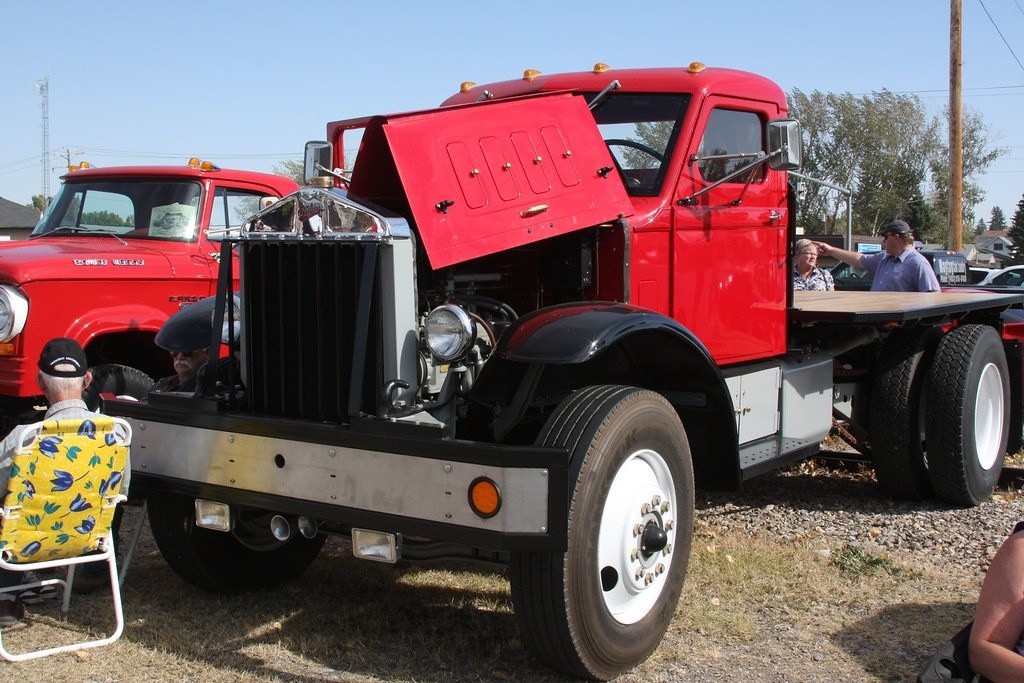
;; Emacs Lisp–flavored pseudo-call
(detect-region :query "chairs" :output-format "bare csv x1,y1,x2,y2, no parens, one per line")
0,418,133,663
119,495,147,587
918,520,1024,683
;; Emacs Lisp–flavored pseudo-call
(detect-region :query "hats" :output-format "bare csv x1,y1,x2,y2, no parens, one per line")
37,338,88,378
877,220,911,236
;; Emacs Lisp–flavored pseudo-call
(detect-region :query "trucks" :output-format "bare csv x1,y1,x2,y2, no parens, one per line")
97,59,1024,683
1,158,299,506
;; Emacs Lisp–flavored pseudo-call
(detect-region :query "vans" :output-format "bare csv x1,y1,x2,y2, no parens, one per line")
830,251,969,292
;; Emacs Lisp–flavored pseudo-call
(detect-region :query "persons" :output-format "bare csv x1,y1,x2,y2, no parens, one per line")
811,220,941,292
969,522,1024,683
793,239,835,291
0,339,212,625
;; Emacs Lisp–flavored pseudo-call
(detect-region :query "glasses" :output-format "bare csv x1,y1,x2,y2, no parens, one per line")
800,251,819,258
168,350,206,358
883,234,901,240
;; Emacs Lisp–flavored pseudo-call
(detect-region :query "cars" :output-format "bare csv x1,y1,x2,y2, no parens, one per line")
935,263,1024,343
